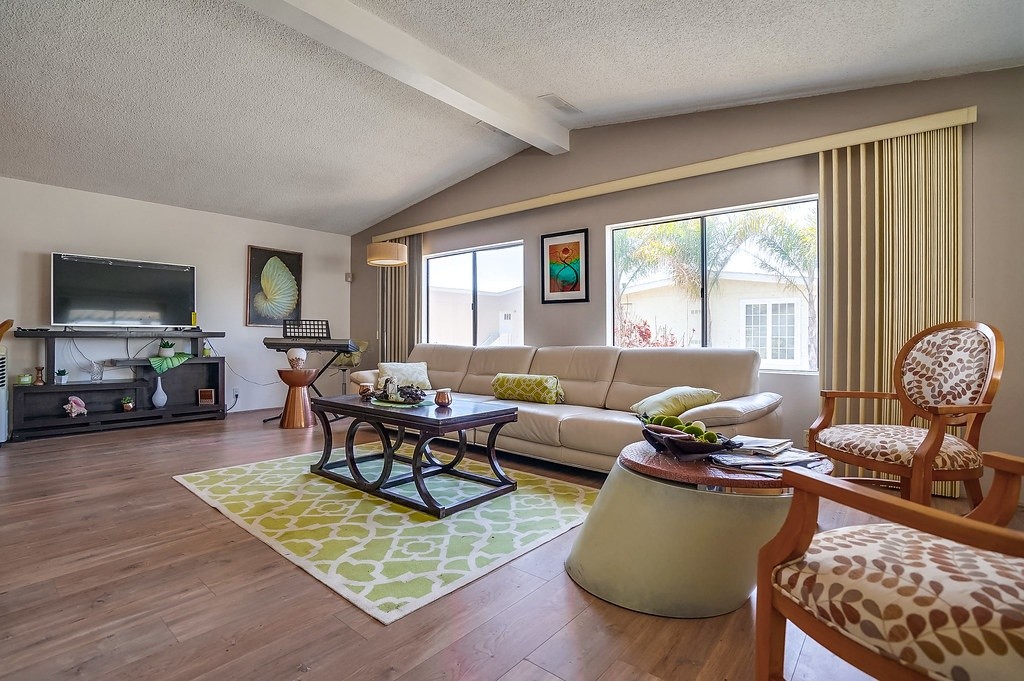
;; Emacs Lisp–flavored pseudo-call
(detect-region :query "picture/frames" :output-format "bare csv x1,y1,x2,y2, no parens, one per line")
247,244,305,329
540,227,590,305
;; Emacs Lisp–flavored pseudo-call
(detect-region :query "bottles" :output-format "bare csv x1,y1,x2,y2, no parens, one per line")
33,366,44,386
151,376,168,409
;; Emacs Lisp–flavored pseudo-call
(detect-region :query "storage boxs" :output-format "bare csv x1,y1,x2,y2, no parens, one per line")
18,373,32,384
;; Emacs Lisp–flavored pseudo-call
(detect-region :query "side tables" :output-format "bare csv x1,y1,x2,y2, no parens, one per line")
562,433,840,619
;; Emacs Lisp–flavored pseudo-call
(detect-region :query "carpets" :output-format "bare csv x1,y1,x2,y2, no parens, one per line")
172,439,600,626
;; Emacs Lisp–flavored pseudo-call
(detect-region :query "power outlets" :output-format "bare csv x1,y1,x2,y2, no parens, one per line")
233,387,240,399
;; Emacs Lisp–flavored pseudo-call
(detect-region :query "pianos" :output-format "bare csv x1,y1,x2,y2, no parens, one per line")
262,336,360,425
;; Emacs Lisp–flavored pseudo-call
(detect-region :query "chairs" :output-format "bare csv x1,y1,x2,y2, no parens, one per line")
324,337,372,396
806,321,1005,512
749,448,1024,681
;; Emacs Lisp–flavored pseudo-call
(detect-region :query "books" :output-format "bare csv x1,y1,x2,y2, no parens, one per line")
708,434,828,479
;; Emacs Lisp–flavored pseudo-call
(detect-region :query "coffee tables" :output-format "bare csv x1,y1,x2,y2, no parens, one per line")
308,389,520,519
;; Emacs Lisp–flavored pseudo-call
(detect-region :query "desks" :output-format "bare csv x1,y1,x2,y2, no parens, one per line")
274,367,320,429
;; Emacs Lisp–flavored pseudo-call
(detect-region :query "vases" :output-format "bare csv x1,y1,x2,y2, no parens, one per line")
287,346,307,369
151,376,168,409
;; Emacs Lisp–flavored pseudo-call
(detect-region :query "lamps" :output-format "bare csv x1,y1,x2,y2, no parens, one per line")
366,240,411,268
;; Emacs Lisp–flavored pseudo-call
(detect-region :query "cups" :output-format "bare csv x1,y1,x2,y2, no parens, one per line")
434,388,452,407
90,360,104,384
359,383,375,395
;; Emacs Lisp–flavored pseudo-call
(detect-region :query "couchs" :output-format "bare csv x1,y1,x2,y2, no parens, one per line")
351,343,785,473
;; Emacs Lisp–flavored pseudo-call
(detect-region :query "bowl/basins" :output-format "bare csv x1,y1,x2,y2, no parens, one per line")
643,424,736,453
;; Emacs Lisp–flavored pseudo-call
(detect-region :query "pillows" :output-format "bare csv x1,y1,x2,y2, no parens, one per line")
630,386,720,423
376,361,432,390
491,370,565,406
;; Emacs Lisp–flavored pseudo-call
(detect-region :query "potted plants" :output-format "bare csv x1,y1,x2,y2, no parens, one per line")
158,337,176,358
54,368,69,384
121,395,136,412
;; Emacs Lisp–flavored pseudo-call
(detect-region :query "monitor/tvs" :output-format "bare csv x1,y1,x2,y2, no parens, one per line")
50,252,197,328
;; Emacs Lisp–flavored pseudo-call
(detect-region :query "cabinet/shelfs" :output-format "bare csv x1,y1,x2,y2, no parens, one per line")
10,328,235,442
